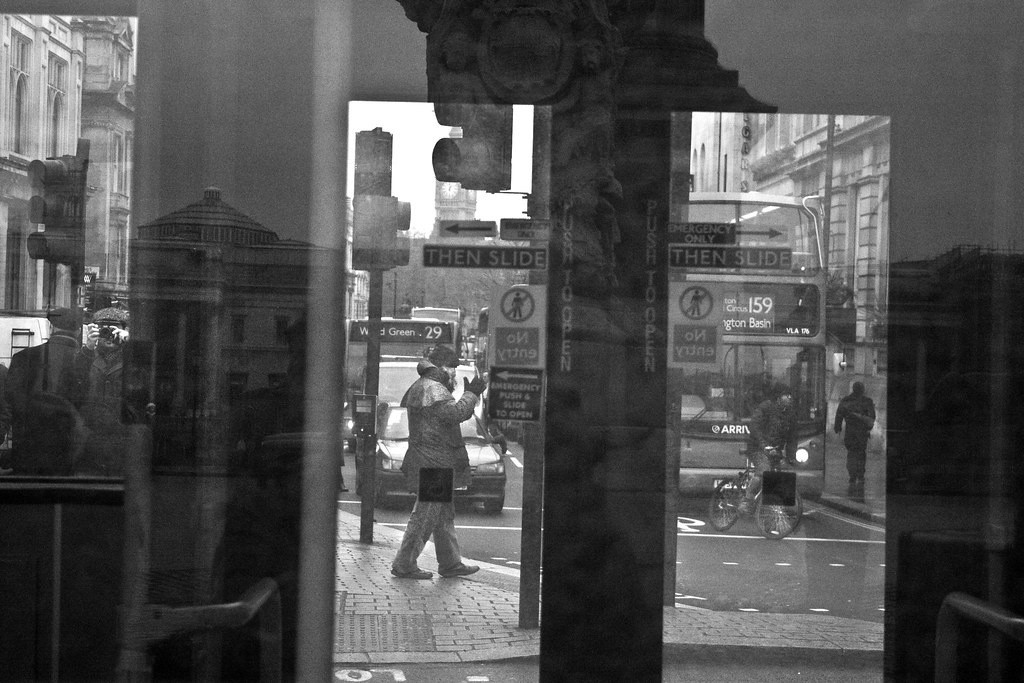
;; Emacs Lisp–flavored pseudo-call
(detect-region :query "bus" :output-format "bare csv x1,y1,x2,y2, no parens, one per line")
463,190,848,497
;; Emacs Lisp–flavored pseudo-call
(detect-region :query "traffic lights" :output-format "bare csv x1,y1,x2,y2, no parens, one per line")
27,154,84,266
431,37,511,194
382,193,409,272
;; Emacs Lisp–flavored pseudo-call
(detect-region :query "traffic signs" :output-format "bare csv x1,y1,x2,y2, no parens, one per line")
437,220,497,238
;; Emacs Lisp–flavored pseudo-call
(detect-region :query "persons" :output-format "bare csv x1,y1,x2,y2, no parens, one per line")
738,386,798,534
834,382,876,481
391,345,486,581
0,305,130,482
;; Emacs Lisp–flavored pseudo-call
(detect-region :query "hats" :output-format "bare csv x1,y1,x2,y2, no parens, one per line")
772,382,792,398
93,307,128,324
47,308,82,330
429,345,461,369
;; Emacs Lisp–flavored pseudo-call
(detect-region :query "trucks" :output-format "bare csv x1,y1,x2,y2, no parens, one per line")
342,307,465,449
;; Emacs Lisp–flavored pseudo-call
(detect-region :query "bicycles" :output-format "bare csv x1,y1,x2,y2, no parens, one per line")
708,448,803,539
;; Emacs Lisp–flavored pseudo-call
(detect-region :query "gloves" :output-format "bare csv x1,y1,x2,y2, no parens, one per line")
463,374,486,397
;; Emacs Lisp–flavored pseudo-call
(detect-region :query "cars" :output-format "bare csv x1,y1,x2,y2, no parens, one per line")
354,402,508,512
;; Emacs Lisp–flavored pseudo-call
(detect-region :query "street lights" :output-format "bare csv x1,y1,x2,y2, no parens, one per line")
346,272,356,317
420,290,426,309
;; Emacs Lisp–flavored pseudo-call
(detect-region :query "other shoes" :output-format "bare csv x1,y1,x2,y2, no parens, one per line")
391,568,433,580
737,496,755,519
439,562,480,578
